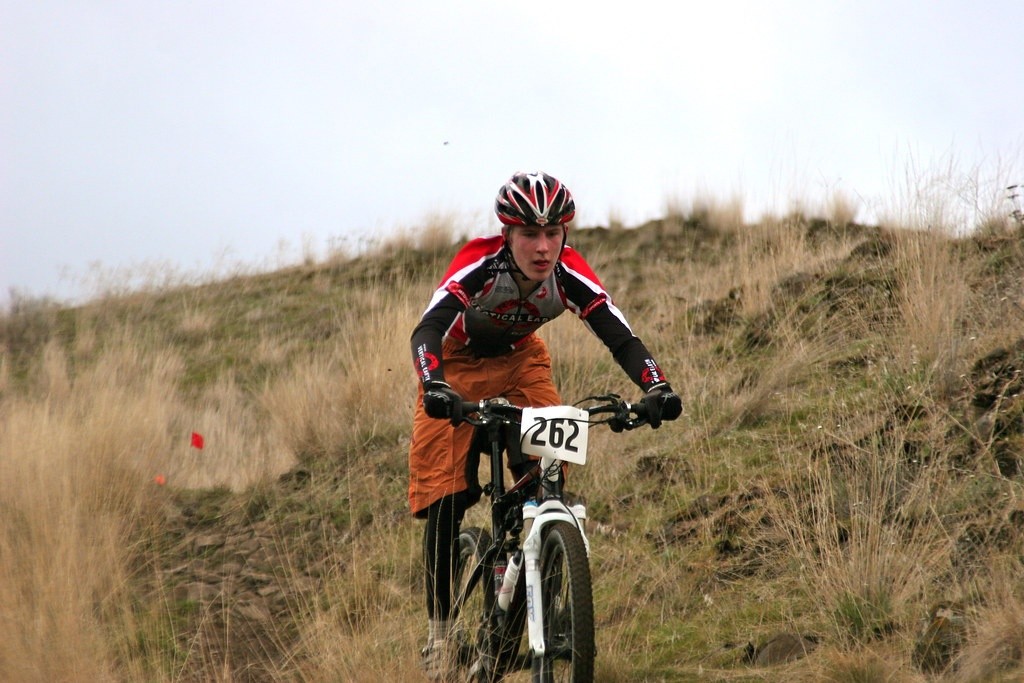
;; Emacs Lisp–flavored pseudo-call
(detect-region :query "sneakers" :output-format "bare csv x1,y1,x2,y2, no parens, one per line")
420,642,451,681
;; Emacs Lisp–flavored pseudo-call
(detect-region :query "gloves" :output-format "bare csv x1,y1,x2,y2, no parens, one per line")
422,380,462,429
640,383,684,429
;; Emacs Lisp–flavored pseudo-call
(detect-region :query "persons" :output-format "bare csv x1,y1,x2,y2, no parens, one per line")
408,173,684,682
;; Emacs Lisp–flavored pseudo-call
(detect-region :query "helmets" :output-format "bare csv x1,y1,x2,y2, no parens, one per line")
494,170,576,226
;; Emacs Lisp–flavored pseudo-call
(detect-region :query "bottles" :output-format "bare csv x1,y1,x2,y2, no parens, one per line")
497,549,525,613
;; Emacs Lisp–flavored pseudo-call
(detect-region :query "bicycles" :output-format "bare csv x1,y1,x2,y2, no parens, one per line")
420,392,674,683
